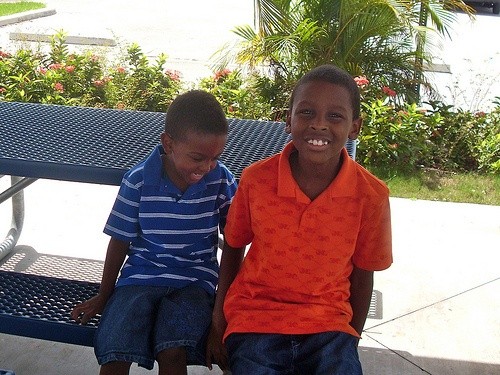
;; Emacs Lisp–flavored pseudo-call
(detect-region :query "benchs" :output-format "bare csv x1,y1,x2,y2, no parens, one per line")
0,270,102,347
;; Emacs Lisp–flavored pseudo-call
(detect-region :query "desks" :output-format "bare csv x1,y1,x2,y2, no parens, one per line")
0,100,356,260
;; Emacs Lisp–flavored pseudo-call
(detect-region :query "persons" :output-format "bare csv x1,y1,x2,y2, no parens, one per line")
206,64,394,375
70,89,239,375
